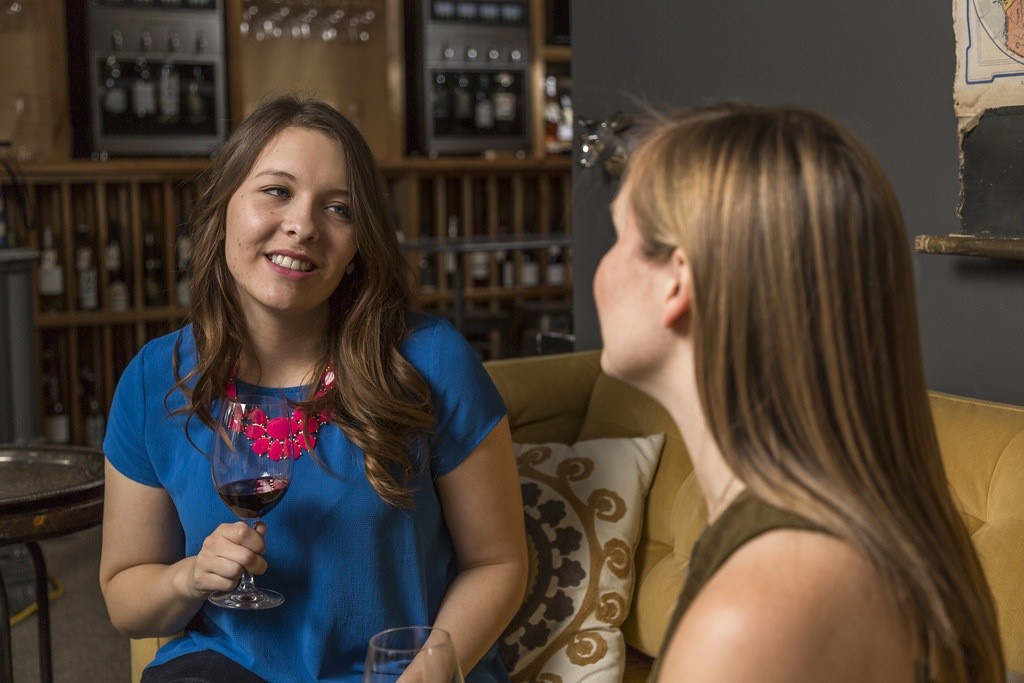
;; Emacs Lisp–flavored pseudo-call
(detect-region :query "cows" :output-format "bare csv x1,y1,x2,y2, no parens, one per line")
574,110,630,178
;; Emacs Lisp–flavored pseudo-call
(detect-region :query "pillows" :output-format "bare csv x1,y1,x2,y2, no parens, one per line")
498,432,666,683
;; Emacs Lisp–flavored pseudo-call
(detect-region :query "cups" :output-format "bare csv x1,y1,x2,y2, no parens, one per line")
364,627,464,683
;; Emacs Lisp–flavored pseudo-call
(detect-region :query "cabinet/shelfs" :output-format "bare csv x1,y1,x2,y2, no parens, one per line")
0,0,574,445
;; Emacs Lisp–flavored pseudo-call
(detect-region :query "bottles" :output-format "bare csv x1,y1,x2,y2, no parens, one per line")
433,73,519,137
103,60,212,134
37,221,193,313
40,368,105,447
542,77,560,146
558,95,573,143
415,207,571,297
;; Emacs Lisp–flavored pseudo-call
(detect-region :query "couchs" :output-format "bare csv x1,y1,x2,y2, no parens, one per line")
130,349,1024,682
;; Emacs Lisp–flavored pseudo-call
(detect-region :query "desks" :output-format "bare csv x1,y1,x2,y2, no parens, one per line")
0,444,105,683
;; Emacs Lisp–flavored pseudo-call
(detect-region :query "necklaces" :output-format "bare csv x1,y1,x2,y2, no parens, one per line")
222,358,336,459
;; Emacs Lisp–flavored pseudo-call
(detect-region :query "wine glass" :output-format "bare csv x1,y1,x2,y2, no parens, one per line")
207,394,291,610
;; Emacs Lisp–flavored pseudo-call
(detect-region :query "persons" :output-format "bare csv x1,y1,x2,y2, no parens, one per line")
591,103,1007,683
97,87,531,683
